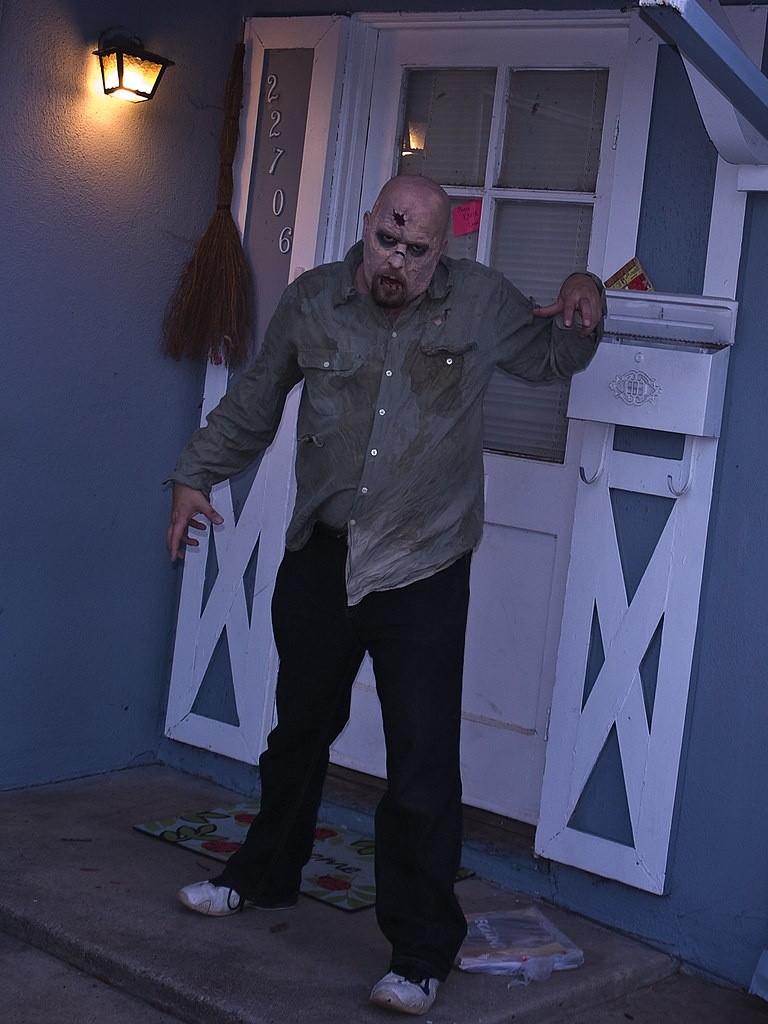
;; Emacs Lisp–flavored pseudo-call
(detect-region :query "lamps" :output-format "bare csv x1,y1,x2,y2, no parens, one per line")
93,26,181,106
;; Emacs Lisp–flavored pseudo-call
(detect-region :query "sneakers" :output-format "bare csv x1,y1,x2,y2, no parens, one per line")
176,878,299,914
372,963,439,1015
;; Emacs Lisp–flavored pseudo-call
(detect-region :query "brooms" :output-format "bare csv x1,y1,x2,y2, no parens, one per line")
159,38,262,372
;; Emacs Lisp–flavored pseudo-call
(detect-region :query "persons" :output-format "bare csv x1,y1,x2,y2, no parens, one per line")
156,175,606,1015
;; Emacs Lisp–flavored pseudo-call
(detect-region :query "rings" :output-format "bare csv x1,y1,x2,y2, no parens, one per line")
578,297,591,308
